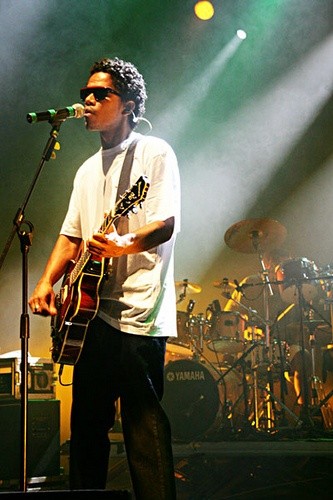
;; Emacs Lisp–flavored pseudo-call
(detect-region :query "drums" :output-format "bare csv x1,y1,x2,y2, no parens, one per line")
236,339,289,378
166,310,205,356
275,257,318,304
161,359,241,443
206,311,245,354
317,265,333,292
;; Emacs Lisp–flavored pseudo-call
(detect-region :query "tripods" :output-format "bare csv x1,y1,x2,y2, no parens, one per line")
178,241,333,441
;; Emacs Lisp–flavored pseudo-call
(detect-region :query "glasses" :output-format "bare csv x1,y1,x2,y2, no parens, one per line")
80,86,125,102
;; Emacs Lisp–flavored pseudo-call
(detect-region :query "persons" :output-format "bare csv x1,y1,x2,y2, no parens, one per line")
28,57,181,500
223,241,320,422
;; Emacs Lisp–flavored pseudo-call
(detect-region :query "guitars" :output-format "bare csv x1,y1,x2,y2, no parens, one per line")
51,175,150,366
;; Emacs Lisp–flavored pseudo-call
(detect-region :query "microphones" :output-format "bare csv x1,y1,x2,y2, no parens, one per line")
234,280,245,298
26,103,84,124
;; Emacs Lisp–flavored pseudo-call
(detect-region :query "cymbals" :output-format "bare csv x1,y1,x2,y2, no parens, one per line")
224,219,287,253
175,282,201,294
286,320,330,329
212,281,237,290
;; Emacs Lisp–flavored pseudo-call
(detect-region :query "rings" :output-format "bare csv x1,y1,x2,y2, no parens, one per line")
35,304,40,309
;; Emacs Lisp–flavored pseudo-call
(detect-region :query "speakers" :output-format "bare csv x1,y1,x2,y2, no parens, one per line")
0,399,60,479
0,489,133,500
